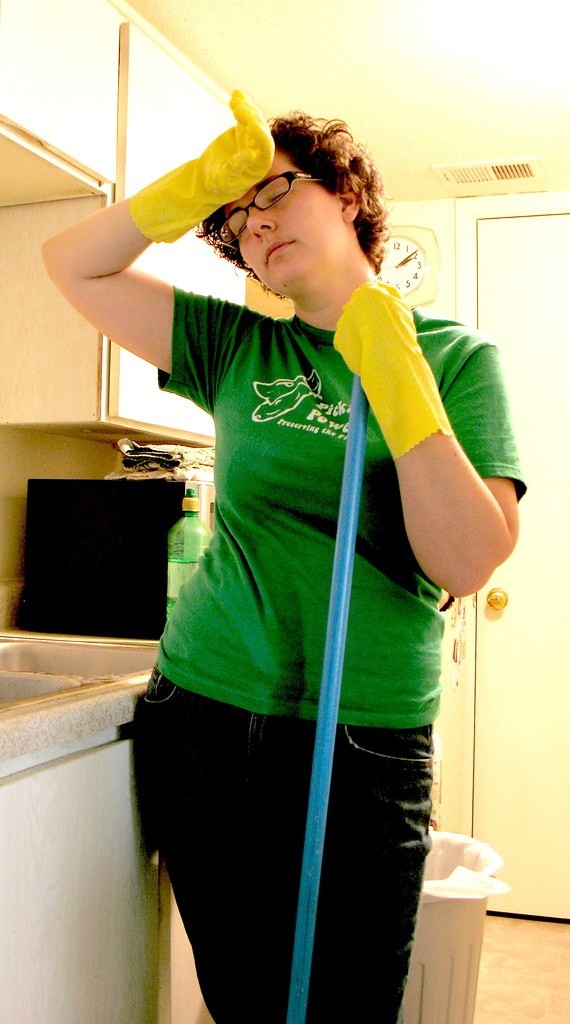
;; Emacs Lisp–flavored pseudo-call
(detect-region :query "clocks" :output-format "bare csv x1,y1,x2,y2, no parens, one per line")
376,223,442,309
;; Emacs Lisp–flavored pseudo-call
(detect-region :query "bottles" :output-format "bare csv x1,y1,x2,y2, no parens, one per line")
166,488,214,621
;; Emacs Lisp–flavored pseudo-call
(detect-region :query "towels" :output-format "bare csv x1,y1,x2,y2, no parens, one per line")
103,437,215,483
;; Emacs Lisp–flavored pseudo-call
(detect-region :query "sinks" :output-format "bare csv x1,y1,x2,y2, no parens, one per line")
1,672,121,711
0,637,159,677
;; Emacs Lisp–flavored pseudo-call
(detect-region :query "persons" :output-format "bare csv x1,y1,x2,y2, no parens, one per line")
42,90,528,1024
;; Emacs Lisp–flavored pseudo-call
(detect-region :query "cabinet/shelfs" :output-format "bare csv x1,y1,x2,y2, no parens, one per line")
0,731,217,1024
0,0,243,441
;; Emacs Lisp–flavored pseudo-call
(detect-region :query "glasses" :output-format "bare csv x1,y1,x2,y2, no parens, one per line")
218,171,322,245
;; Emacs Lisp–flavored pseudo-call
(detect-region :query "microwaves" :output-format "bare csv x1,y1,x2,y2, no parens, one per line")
19,478,216,641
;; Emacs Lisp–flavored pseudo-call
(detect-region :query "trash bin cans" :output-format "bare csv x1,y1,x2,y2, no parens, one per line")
396,829,502,1024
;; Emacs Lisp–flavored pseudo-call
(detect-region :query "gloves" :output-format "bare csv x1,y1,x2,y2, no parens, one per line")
333,280,455,460
129,88,275,244
